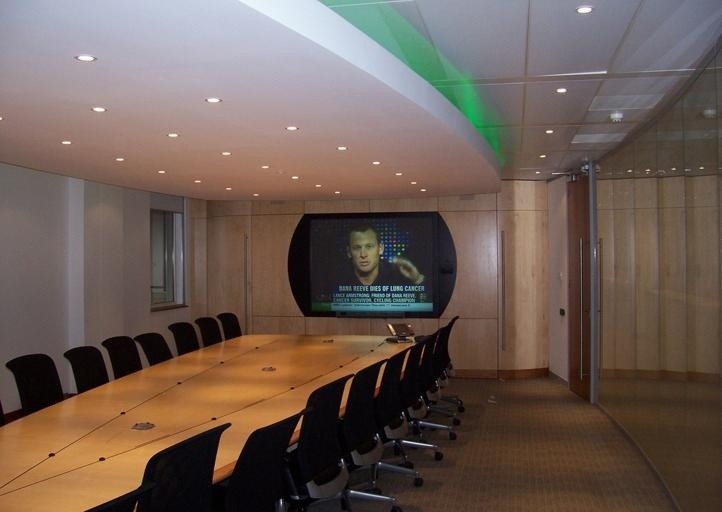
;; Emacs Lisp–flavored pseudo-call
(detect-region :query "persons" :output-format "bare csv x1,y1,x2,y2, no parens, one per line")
318,221,433,303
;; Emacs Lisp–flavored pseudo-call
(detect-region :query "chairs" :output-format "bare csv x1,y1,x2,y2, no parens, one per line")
194,316,223,349
100,335,143,381
5,353,65,418
309,359,401,512
377,334,444,470
353,346,425,493
279,372,355,512
130,423,231,512
416,318,462,428
169,321,200,357
215,409,304,512
134,332,174,366
215,312,242,340
432,316,464,410
403,324,458,445
62,345,110,394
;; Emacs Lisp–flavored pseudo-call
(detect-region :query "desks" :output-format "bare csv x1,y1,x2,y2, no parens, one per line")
1,334,420,511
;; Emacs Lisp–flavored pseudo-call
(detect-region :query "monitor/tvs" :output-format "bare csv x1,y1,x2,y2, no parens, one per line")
304,211,439,317
